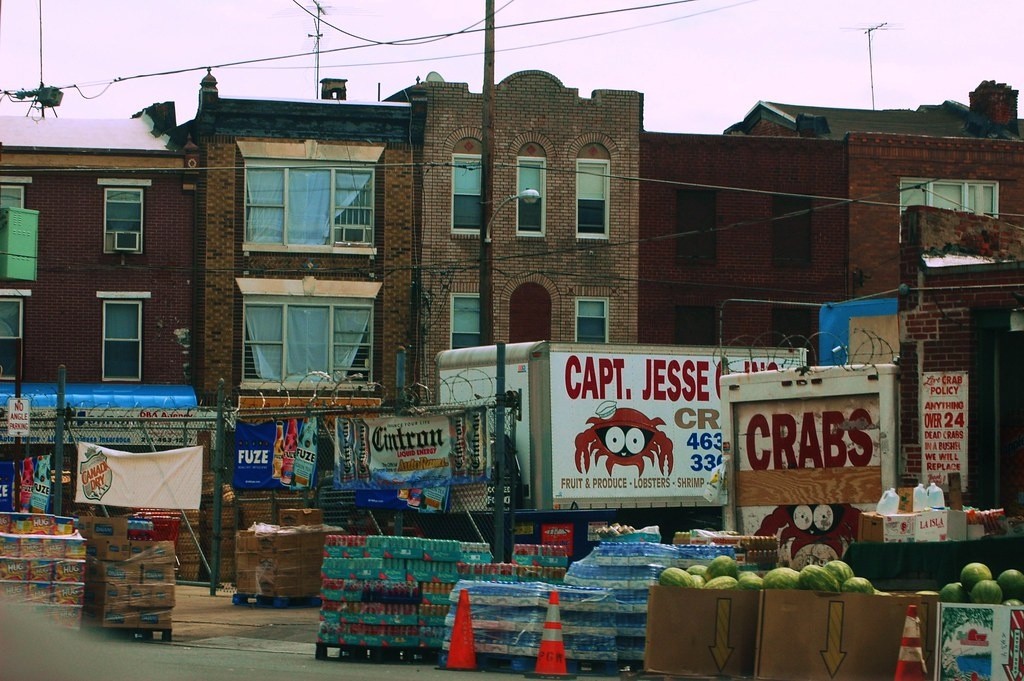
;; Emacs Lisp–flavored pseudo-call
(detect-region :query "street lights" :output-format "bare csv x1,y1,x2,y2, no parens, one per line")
479,188,541,345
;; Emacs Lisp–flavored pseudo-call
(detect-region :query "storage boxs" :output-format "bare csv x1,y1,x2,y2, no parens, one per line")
939,602,1024,681
79,517,176,629
645,584,938,681
234,508,563,647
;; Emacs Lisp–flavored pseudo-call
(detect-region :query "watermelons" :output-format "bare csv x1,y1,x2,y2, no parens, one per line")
659,556,1024,606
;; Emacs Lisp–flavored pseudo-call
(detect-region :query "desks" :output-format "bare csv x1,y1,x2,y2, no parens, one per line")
858,511,967,543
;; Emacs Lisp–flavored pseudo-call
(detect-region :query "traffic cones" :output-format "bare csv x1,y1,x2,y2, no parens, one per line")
896,604,929,680
432,588,488,673
522,591,578,680
